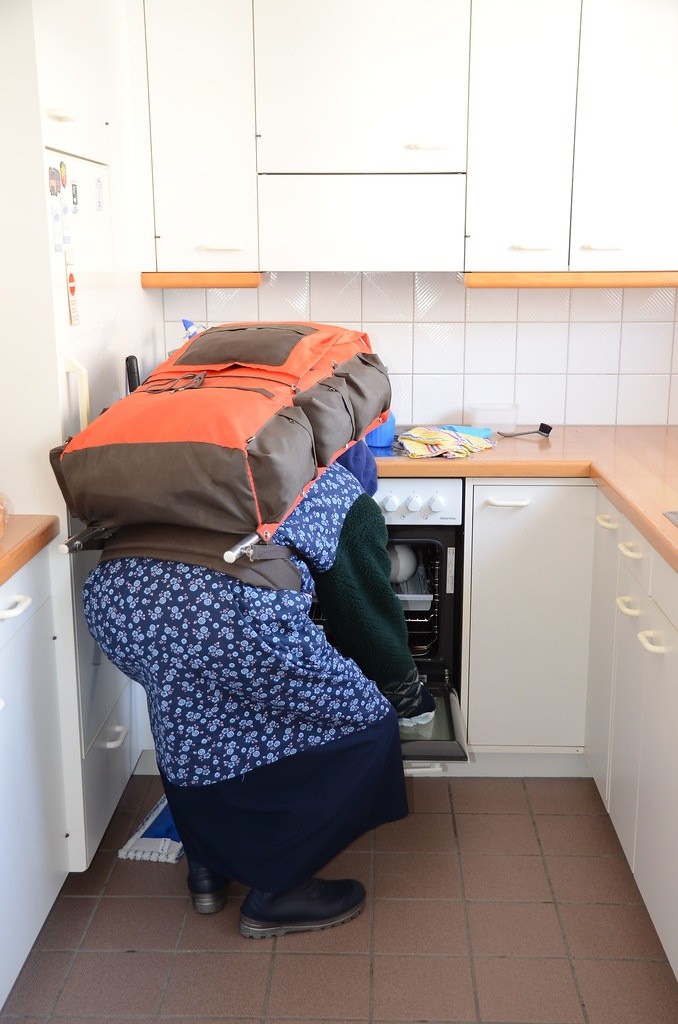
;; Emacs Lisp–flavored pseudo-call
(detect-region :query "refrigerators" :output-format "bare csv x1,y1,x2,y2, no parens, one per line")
1,1,143,874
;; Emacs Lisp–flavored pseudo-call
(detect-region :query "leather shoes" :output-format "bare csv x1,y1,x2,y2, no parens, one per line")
239,877,367,939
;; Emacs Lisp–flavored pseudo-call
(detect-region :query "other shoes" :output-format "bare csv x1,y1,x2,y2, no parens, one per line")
190,881,228,913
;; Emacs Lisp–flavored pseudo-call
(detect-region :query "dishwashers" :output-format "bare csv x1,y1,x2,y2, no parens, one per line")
368,477,471,762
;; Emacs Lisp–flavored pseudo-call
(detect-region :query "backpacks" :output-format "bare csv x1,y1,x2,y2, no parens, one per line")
48,319,395,532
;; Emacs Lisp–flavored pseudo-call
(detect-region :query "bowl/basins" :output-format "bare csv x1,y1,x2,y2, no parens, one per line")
389,544,417,583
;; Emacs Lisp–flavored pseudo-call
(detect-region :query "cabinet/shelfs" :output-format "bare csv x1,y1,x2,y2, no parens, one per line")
50,547,145,872
144,1,677,272
449,477,678,979
0,513,79,1013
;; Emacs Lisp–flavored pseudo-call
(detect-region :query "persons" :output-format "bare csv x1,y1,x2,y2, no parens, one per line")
81,441,435,936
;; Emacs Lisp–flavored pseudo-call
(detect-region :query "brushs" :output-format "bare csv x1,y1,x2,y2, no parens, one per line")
497,422,554,438
